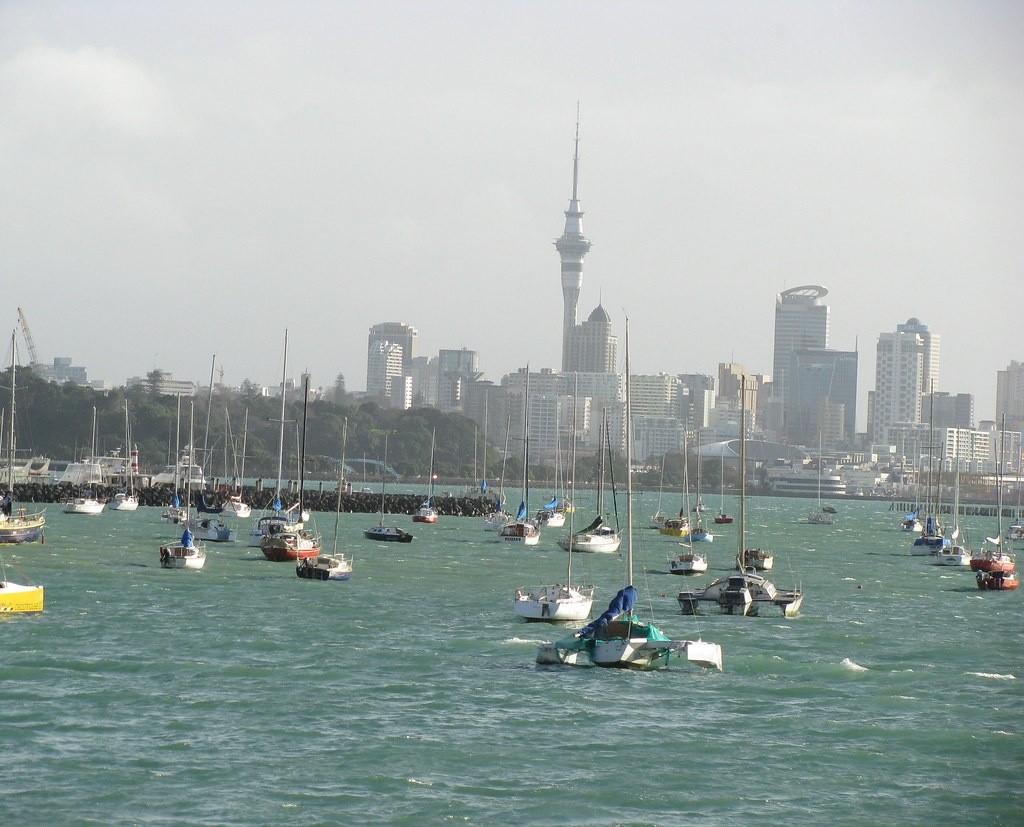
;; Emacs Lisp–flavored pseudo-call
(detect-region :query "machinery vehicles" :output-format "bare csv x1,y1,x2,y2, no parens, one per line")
13,307,50,381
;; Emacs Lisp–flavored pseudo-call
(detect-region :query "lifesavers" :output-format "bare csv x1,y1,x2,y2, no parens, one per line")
120,468,126,474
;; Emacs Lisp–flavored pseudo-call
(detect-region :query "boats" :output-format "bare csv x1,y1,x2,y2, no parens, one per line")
734,547,775,570
0,551,48,616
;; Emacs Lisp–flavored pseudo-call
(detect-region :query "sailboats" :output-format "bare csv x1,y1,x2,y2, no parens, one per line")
664,418,708,574
295,416,353,580
158,399,207,570
1,324,712,561
676,373,805,618
512,370,595,620
533,314,723,671
799,376,1023,592
